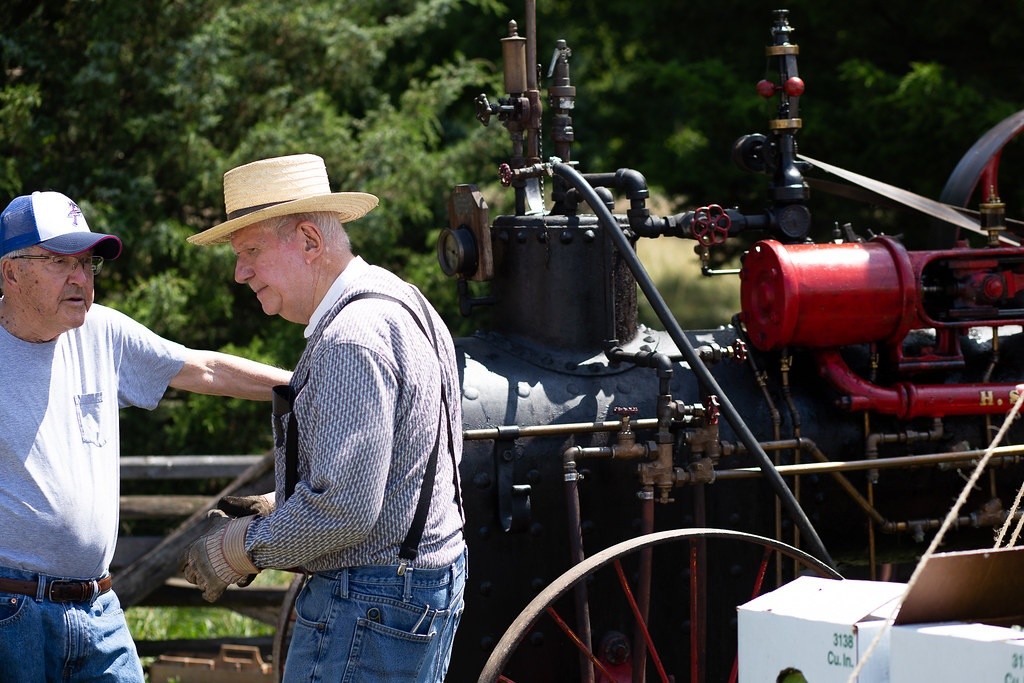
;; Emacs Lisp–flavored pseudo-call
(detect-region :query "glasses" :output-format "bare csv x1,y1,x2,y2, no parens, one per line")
12,255,104,277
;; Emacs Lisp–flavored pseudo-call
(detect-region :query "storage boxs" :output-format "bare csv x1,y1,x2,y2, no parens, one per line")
734,574,909,683
886,544,1024,681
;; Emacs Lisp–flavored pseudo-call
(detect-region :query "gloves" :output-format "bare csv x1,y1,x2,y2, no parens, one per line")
177,492,276,602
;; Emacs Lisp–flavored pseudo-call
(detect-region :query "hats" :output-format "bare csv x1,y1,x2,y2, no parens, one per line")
186,153,379,248
0,191,122,261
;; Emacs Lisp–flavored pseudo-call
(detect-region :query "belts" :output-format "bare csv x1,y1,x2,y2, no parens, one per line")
1,574,112,602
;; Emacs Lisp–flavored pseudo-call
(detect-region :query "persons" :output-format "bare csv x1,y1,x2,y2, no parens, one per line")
1,190,302,683
178,200,473,683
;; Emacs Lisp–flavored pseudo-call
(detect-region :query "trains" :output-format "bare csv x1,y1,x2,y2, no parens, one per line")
270,1,1023,683
